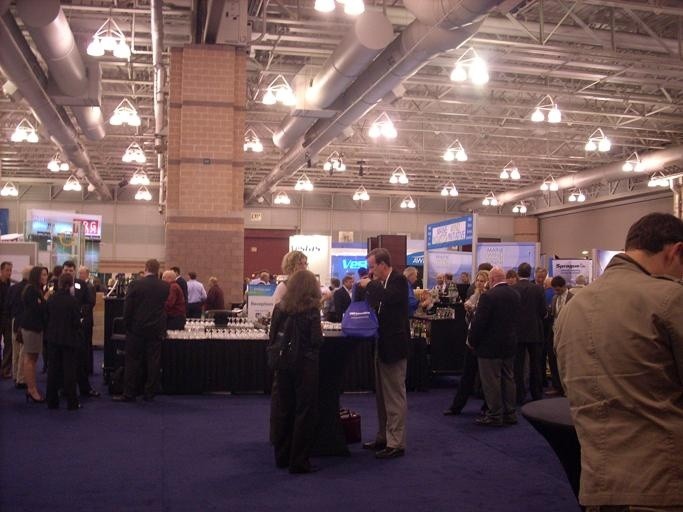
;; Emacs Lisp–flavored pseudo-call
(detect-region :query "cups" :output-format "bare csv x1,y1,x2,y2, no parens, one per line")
322,320,340,331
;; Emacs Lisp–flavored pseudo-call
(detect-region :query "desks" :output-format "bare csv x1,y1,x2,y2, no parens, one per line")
162,329,428,393
522,398,586,512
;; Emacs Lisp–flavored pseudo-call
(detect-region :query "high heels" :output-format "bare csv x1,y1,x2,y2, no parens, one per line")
26,389,46,403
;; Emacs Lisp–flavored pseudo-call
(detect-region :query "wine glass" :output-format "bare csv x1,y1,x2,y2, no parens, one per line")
166,317,269,341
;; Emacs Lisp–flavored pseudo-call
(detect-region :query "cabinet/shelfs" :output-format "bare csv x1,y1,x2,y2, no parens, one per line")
408,283,472,377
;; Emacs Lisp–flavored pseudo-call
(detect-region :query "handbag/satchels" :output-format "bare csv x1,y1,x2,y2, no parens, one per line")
269,339,308,377
108,367,143,396
337,300,379,337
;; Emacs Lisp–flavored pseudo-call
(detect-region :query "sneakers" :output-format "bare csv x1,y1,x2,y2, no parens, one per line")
444,409,453,415
504,411,519,424
473,414,503,426
89,389,101,397
15,383,26,389
545,387,559,396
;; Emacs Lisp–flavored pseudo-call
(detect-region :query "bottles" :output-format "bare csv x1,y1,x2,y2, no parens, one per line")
114,272,131,297
410,287,430,344
435,283,458,318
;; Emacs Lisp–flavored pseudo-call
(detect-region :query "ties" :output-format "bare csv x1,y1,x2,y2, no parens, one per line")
558,295,562,311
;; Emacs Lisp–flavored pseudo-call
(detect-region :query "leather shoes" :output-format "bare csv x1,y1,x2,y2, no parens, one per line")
289,464,320,472
363,439,387,448
374,447,404,458
111,393,135,402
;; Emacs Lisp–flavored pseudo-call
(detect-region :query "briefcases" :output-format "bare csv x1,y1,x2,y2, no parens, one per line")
339,408,362,443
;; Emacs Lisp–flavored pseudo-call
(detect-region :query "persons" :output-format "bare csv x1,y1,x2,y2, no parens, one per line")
0,258,226,412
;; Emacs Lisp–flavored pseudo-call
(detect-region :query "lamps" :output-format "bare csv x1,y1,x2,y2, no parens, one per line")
261,75,296,106
400,195,414,208
323,152,346,172
47,154,71,172
585,128,612,152
10,118,38,142
274,189,289,204
482,192,497,207
63,175,81,191
512,201,527,213
499,160,520,180
122,142,146,163
568,188,586,202
540,174,559,191
443,139,468,162
109,99,140,127
531,95,561,123
85,18,131,59
648,170,668,187
314,2,365,16
130,168,152,201
1,182,19,196
389,166,407,183
353,184,369,200
242,130,263,153
441,181,458,197
450,47,489,85
295,173,313,190
623,152,645,172
368,112,397,138
